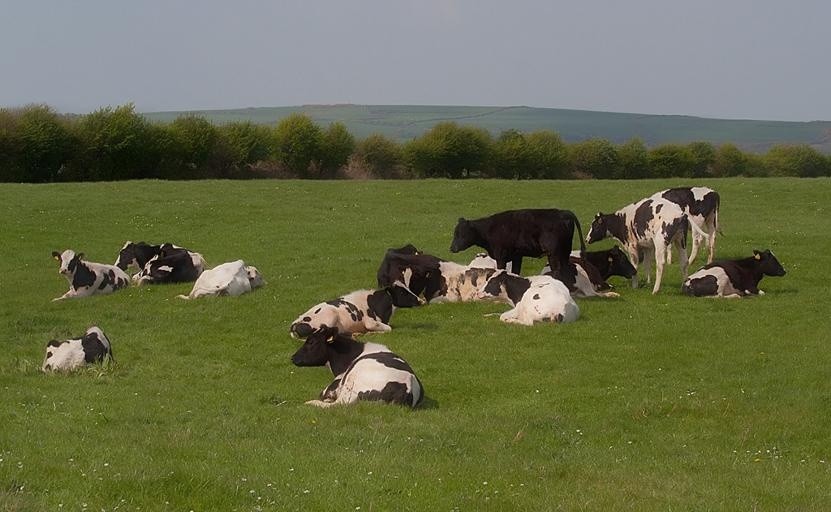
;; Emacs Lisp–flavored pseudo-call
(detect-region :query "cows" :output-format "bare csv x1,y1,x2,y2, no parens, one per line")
678,245,788,300
376,208,638,330
288,278,426,342
42,323,116,376
584,185,725,297
49,240,263,305
289,324,426,411
